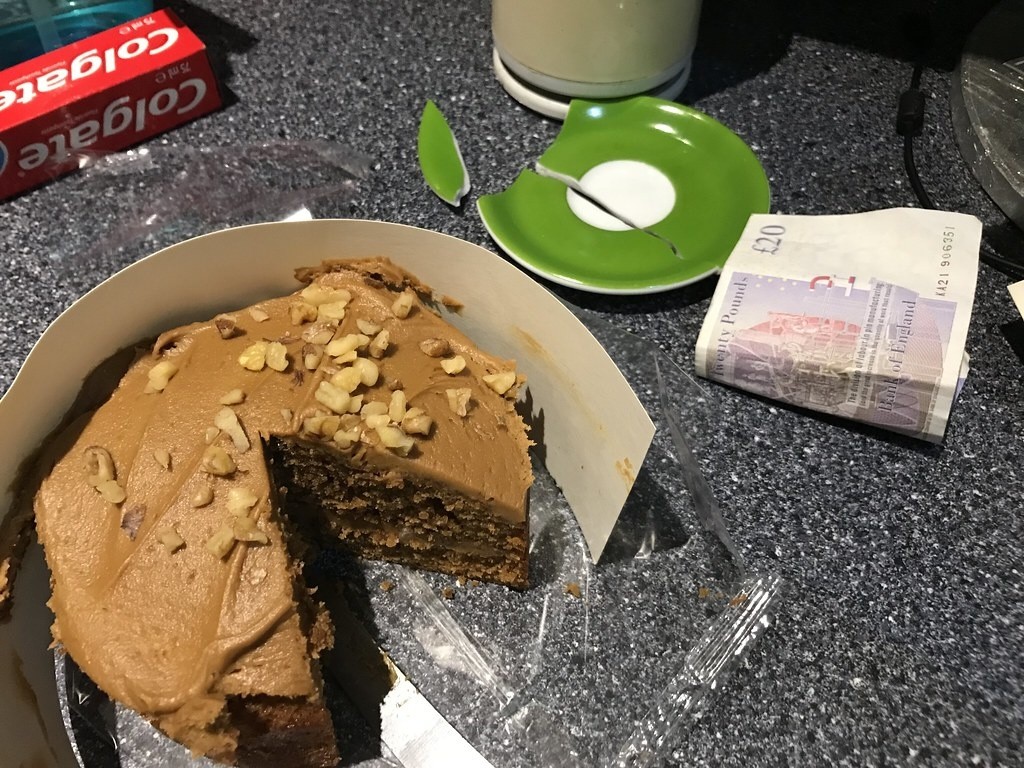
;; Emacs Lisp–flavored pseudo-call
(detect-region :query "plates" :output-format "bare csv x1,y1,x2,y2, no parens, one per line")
417,89,772,296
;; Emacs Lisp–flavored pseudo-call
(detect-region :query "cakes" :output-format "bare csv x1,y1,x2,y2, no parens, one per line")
32,266,531,768
